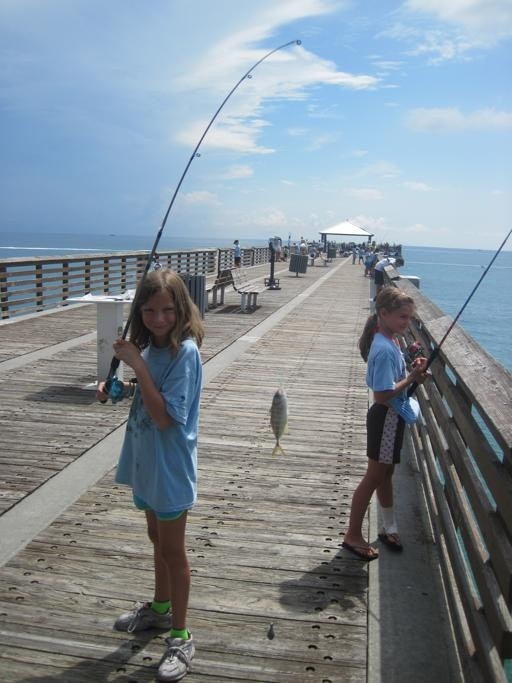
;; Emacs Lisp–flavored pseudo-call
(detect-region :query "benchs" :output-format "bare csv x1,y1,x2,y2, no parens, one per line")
206,266,266,313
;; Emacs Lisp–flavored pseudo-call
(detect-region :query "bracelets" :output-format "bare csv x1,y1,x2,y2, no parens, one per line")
128,380,135,398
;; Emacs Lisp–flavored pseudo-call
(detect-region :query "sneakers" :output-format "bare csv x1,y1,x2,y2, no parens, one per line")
114,602,173,632
158,629,195,681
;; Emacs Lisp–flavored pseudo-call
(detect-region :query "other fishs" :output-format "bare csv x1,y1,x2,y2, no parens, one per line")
269,388,290,457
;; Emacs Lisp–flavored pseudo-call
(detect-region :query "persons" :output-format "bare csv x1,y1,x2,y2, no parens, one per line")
232,239,242,268
343,284,428,559
373,256,404,301
95,270,204,682
268,235,378,277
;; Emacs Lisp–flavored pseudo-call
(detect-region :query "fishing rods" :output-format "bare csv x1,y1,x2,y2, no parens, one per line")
407,230,511,397
98,39,301,402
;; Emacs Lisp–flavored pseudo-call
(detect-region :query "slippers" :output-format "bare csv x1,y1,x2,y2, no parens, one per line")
379,532,403,552
343,541,379,559
